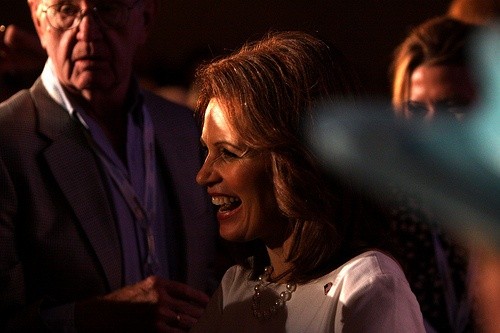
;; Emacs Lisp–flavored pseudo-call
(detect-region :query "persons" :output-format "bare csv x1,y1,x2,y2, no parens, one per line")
192,31,430,333
0,0,239,333
381,14,500,333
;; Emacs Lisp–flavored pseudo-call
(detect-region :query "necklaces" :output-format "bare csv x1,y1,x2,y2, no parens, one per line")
248,266,297,319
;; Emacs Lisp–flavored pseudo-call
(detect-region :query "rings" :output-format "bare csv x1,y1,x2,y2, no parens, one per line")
170,312,181,328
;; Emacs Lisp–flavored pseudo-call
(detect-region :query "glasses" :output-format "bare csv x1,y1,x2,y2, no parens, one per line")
41,2,132,32
